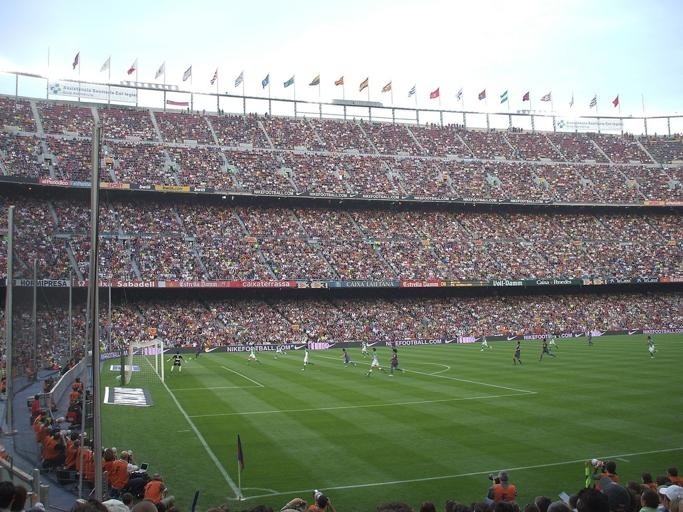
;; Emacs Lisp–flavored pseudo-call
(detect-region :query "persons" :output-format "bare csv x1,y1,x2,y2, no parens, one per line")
168,351,188,374
0,353,179,511
206,453,683,512
388,348,406,376
0,94,683,368
364,347,389,376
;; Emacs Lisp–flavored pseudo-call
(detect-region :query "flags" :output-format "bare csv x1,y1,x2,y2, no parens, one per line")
478,88,487,101
309,73,320,86
500,90,509,104
359,77,369,93
99,56,111,74
408,84,417,98
262,72,270,89
381,80,391,93
334,76,344,86
155,62,166,79
540,91,553,103
234,69,244,88
458,88,467,101
430,87,439,99
210,67,218,85
236,433,247,472
567,91,574,108
71,51,81,71
522,91,530,101
283,75,295,88
127,57,138,75
182,65,192,82
